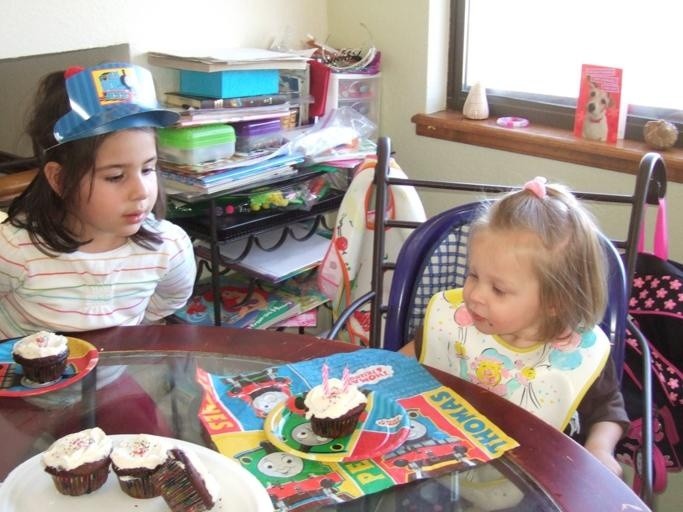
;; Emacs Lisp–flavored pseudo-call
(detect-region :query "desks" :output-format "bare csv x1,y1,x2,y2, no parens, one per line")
0,325,653,512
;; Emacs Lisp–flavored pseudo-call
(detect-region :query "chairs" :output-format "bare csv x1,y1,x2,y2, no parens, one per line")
379,200,627,428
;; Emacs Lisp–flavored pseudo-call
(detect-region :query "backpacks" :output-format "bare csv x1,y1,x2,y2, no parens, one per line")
614,198,682,493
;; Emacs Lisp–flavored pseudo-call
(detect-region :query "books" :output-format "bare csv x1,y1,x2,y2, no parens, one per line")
143,49,306,128
157,150,304,208
174,221,332,329
573,63,629,143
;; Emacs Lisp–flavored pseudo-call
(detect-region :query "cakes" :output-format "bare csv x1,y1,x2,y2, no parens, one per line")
304,378,368,437
110,433,174,499
11,330,70,382
39,426,112,496
152,449,218,512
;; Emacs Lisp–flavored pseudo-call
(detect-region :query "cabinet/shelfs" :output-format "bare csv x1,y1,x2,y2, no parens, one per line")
159,159,361,335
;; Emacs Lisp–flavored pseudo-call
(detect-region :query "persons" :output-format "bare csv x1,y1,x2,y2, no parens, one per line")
395,176,630,477
0,60,197,341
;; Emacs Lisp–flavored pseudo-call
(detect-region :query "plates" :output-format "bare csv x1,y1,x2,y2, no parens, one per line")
263,389,411,463
0,433,275,512
0,336,98,397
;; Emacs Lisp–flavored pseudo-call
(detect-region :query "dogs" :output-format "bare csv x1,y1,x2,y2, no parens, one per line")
581,75,615,143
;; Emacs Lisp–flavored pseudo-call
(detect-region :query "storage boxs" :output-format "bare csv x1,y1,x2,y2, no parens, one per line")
313,72,381,124
